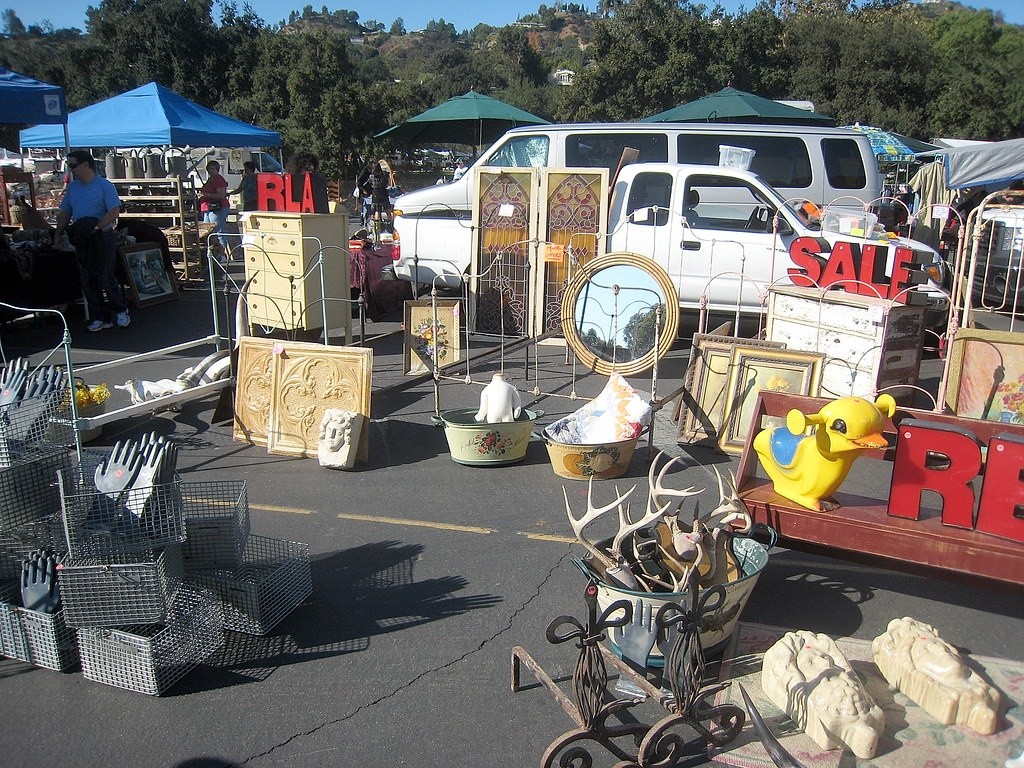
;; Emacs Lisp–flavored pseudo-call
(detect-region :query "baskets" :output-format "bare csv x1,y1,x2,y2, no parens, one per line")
0,387,314,695
51,376,111,445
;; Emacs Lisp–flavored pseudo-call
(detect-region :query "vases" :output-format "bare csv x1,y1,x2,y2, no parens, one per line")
48,403,105,445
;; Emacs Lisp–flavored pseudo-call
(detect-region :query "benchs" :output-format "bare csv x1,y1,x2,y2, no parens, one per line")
725,390,1024,601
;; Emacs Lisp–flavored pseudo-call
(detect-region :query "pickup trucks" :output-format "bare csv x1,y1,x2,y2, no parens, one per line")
392,162,949,349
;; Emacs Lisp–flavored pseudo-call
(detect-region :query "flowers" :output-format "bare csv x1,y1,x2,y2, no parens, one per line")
58,380,112,415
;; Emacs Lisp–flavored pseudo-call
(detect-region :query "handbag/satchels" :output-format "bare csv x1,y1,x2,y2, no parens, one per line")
207,201,222,211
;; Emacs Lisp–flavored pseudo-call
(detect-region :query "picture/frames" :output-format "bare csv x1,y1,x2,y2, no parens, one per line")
943,327,1024,465
232,335,320,448
402,300,461,376
266,342,373,465
714,342,828,461
119,241,181,310
675,332,788,448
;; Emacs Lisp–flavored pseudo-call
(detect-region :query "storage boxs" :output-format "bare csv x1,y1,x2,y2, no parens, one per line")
719,144,756,171
822,206,879,239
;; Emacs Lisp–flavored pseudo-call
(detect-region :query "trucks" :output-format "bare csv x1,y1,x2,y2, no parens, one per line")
117,146,287,195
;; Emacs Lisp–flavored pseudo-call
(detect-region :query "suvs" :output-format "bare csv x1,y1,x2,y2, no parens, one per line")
964,202,1024,317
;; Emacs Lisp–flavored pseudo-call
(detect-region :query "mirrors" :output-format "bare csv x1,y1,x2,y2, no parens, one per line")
560,251,681,377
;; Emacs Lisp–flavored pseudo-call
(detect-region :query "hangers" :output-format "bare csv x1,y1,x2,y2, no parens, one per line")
919,152,942,168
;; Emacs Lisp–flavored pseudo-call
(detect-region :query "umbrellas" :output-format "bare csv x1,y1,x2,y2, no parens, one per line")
623,86,837,128
372,88,554,156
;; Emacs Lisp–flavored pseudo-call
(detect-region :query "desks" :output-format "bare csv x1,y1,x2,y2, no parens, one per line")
0,247,75,327
349,243,401,323
11,237,128,321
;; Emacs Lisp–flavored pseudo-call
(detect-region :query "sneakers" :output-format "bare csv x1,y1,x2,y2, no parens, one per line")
117,308,131,328
86,320,114,331
220,256,226,263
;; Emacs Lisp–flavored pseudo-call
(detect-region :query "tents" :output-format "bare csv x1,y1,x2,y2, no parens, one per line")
17,80,287,226
0,66,70,150
838,122,1024,218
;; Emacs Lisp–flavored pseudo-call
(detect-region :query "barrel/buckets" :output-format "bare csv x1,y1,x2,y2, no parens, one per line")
569,523,779,667
531,421,651,481
430,408,545,467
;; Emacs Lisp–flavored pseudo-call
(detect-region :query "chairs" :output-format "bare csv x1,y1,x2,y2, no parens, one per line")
685,190,700,225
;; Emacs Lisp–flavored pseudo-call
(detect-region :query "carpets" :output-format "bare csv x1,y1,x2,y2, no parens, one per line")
706,620,1024,768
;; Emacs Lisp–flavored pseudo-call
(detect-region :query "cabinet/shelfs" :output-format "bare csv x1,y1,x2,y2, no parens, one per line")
107,174,203,290
764,283,929,447
237,210,352,345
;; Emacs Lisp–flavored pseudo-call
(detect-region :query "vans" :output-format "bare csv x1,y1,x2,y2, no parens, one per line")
394,123,880,266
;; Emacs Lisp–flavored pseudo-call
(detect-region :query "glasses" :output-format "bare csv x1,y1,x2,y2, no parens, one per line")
67,160,85,169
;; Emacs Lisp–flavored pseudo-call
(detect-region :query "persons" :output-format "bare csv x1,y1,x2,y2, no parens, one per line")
890,184,910,211
356,158,469,228
195,160,235,260
792,198,827,222
53,150,132,332
227,161,258,211
58,158,73,196
